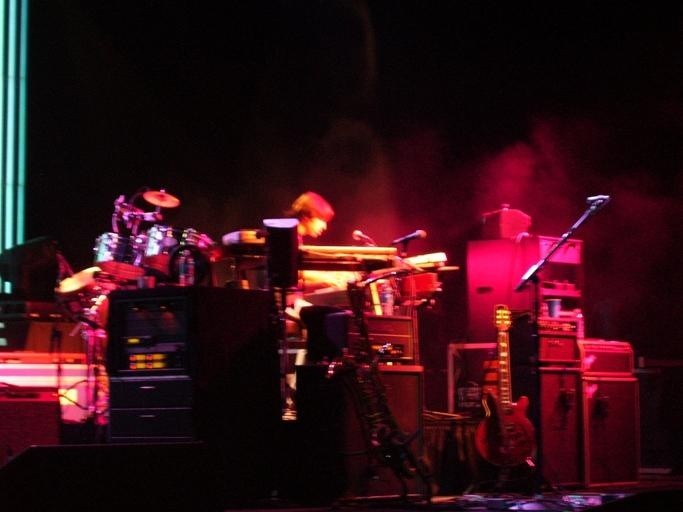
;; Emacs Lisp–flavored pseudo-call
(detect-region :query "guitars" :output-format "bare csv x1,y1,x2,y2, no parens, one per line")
470,304,540,468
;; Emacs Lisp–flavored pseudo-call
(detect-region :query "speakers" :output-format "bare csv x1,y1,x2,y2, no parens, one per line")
0,442,225,512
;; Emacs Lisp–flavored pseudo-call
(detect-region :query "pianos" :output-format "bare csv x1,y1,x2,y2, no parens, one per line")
209,245,405,288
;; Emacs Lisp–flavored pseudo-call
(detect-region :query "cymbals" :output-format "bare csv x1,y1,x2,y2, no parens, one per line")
141,190,181,209
53,260,147,294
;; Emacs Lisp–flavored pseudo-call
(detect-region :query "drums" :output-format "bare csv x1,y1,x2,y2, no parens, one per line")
348,270,403,311
402,273,439,294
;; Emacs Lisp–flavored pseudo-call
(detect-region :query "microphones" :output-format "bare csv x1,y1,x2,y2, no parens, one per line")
352,229,374,245
393,228,426,245
587,196,610,203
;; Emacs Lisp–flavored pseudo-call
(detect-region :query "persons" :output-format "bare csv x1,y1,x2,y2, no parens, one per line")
279,191,336,421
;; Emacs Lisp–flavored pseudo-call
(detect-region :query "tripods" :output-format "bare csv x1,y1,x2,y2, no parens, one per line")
506,283,574,512
62,320,111,427
